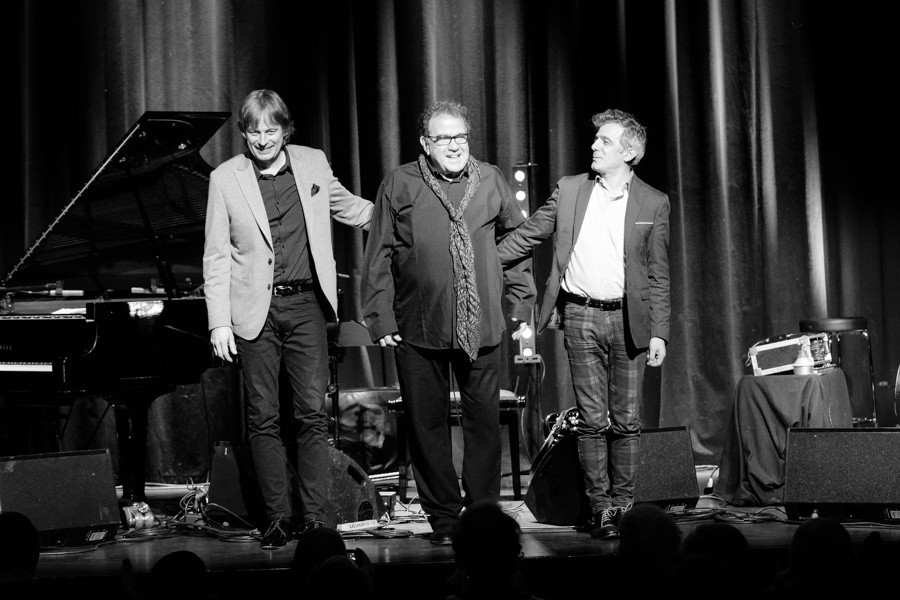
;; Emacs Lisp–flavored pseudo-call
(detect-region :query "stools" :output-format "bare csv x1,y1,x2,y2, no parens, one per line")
389,388,526,501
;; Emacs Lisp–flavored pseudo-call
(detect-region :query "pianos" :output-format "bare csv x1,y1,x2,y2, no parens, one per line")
0,110,230,504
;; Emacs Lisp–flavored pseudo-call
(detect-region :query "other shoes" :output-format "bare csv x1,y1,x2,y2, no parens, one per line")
260,520,291,549
304,522,322,535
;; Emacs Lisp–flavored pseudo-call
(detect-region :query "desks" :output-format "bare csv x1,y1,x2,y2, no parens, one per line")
713,368,852,507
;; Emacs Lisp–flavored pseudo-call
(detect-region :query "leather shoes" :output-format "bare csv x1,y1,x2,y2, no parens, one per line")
432,532,454,545
594,502,634,539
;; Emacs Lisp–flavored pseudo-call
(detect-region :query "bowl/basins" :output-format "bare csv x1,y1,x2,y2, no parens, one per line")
792,365,812,376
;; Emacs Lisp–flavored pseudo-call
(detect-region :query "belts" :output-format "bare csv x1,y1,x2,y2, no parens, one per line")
567,294,626,311
273,284,312,297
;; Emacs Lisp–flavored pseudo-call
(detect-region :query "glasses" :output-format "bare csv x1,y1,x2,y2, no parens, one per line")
424,133,469,145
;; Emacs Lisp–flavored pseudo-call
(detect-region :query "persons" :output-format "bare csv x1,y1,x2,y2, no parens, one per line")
361,101,537,546
202,89,376,548
497,109,671,540
2,504,899,600
